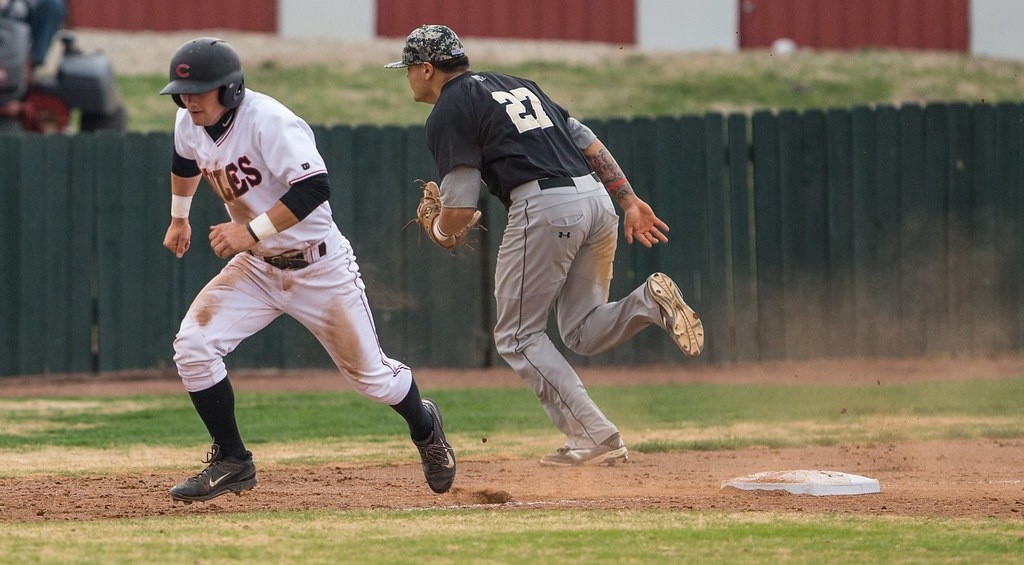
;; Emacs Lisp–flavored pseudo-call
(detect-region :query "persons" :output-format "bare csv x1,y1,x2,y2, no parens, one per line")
0,0,128,136
384,24,704,468
159,38,457,505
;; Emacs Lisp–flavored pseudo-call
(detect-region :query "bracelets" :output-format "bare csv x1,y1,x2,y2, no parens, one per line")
246,213,278,243
433,220,449,242
170,194,193,218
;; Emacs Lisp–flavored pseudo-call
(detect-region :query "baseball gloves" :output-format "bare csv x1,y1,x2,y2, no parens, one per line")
416,180,483,253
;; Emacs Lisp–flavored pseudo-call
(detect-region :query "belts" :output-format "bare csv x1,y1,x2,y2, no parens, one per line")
249,242,326,270
538,171,601,190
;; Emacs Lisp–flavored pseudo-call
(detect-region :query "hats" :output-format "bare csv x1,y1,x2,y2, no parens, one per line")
384,24,465,69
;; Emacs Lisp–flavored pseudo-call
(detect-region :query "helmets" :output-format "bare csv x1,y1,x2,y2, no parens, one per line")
160,37,245,109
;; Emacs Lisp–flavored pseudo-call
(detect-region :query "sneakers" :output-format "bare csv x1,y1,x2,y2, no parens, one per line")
539,429,629,468
406,399,456,494
644,273,704,358
170,436,257,506
